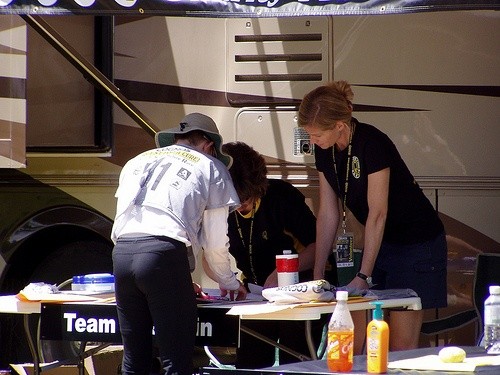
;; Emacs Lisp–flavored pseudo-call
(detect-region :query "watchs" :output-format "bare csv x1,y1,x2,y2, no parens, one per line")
358,273,372,283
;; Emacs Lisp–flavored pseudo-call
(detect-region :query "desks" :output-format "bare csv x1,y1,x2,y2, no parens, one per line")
0,286,500,375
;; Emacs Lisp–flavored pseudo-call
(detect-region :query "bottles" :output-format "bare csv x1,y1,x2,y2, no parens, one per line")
327,290,354,373
483,285,500,353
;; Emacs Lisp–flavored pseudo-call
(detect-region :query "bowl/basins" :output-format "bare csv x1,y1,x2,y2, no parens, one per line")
72,276,115,291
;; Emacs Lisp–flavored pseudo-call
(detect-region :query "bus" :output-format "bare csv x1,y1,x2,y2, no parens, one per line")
0,1,500,375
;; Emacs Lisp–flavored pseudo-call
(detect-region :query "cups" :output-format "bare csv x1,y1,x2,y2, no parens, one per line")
275,250,300,287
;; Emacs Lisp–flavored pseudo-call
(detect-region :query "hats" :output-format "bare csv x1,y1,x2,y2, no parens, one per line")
155,113,233,170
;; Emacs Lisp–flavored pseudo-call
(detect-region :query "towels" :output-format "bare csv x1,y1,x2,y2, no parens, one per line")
261,279,418,304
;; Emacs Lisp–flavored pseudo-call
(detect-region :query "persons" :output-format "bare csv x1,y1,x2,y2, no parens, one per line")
297,80,448,355
219,142,338,371
111,113,247,375
